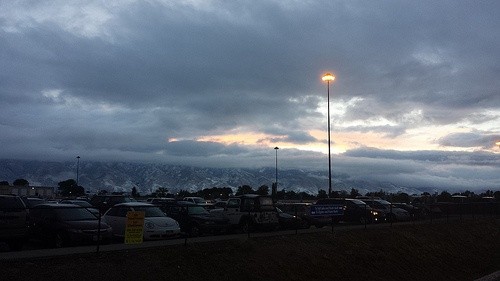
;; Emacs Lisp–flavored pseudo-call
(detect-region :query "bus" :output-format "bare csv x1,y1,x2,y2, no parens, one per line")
450,195,467,202
482,197,497,202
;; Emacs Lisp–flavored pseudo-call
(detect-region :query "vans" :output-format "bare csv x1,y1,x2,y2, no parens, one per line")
318,197,383,226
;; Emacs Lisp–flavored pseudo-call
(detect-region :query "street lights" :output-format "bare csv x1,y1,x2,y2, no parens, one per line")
274,147,281,183
322,73,335,198
76,156,81,186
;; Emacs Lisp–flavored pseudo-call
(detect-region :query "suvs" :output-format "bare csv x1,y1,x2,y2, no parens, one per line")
209,196,282,234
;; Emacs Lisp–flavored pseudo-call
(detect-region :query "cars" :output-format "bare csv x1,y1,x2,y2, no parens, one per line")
279,208,303,229
393,203,423,222
361,198,410,224
30,190,242,212
31,205,113,248
0,194,32,248
101,202,183,244
162,201,226,237
281,202,331,227
